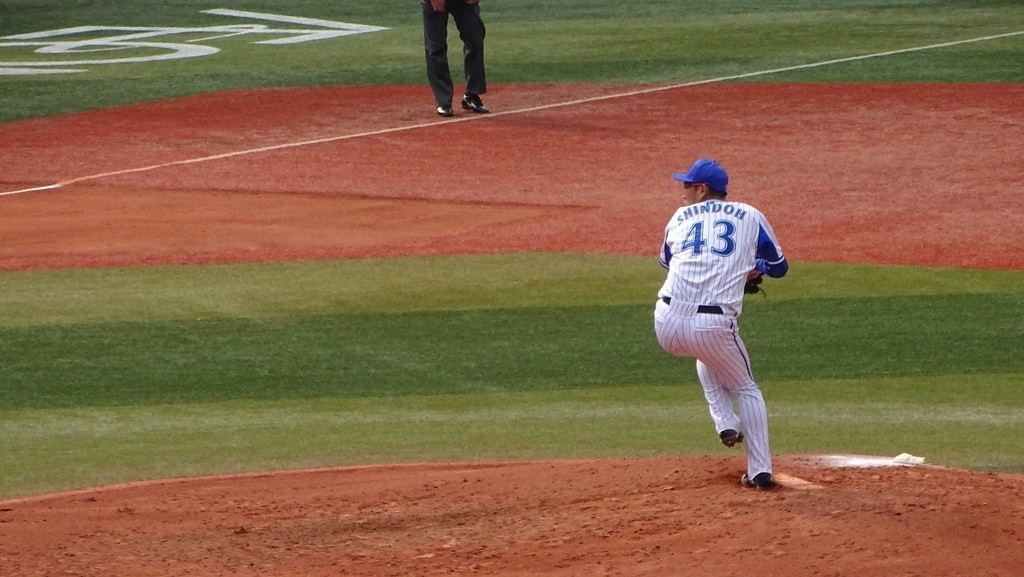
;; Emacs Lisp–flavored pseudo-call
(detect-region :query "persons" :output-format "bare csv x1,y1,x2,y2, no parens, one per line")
654,159,788,490
420,0,489,117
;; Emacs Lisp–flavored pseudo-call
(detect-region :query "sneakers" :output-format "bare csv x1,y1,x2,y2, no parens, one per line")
437,105,453,117
462,94,489,113
741,472,772,490
720,430,743,446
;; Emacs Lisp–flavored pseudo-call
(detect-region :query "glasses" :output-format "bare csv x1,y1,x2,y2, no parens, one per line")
683,182,711,190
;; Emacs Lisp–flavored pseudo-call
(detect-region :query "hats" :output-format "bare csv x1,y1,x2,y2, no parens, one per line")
673,158,728,191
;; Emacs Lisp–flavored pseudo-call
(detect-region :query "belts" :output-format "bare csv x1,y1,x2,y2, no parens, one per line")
663,296,723,314
